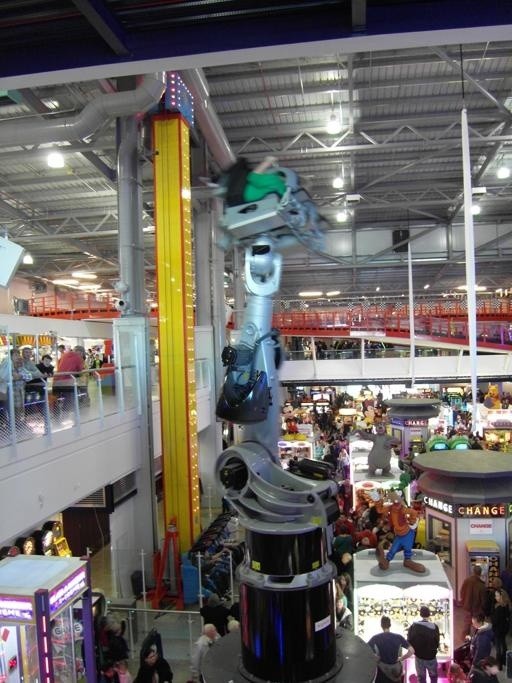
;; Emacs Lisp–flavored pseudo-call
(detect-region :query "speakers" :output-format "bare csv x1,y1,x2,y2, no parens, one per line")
393,230,408,252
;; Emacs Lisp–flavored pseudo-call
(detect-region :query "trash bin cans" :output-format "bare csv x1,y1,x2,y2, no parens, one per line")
107,599,138,645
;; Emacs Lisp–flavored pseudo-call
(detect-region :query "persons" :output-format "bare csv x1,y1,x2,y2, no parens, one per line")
311,390,511,683
201,153,297,206
0,339,114,440
80,514,245,682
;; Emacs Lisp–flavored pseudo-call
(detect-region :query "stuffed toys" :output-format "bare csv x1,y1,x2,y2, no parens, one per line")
281,401,307,441
483,382,501,409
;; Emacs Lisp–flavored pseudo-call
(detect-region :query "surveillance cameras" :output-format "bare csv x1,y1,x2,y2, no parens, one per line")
115,299,127,311
